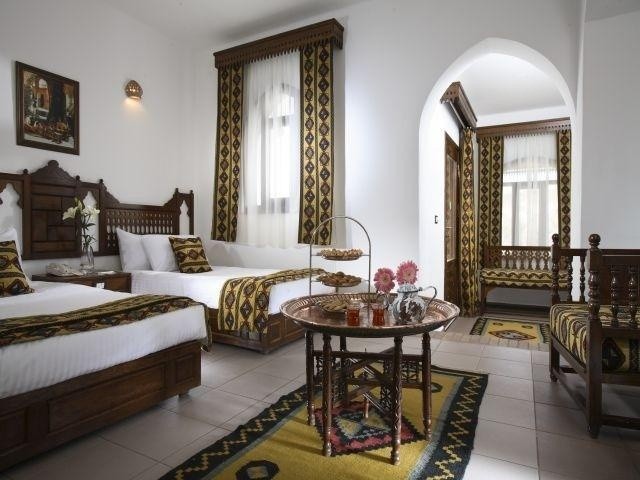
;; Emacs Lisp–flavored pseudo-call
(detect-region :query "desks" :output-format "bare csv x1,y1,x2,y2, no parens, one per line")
279,293,460,466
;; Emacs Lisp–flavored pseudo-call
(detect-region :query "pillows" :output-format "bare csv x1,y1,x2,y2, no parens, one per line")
170,236,211,273
116,228,151,271
0,240,35,298
142,235,179,273
0,229,32,287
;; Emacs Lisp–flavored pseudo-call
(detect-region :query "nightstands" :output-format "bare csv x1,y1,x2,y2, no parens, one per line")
32,269,131,293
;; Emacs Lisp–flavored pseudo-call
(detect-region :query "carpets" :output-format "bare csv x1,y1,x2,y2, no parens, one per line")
469,317,551,343
160,358,488,480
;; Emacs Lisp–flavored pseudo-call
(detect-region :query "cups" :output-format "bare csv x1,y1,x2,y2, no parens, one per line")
346,318,361,328
371,291,390,310
372,316,385,327
372,304,384,316
345,304,360,318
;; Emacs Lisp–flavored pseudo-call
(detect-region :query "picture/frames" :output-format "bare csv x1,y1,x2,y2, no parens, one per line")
15,60,80,156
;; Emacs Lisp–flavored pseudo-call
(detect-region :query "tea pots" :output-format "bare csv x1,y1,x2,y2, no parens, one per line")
378,284,438,327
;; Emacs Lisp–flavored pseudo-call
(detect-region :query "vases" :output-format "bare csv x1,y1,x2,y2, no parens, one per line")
82,245,94,272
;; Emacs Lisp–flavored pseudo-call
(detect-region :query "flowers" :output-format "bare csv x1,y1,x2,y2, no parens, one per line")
361,260,419,302
63,198,100,262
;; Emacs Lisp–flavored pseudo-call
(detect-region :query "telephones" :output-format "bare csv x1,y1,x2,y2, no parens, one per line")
46,263,84,277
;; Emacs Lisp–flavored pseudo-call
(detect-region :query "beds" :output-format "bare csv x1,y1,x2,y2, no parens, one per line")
131,265,334,355
0,281,212,470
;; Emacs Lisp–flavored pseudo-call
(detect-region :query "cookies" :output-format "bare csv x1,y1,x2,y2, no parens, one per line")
318,272,361,285
317,301,346,311
319,249,362,260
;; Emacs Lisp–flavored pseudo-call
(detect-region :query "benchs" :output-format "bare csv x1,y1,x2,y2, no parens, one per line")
479,245,568,316
549,234,640,439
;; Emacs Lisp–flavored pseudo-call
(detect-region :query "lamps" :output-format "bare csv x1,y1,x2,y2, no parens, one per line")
125,81,143,99
